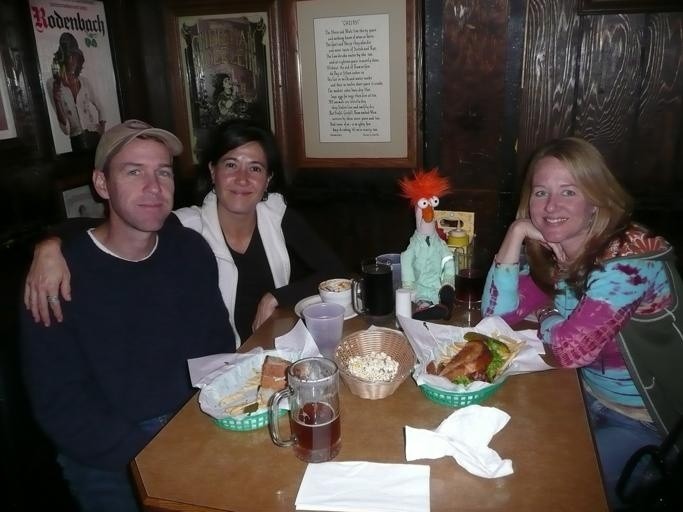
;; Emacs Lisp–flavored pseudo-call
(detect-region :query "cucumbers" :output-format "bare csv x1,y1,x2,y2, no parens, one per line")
463,332,509,353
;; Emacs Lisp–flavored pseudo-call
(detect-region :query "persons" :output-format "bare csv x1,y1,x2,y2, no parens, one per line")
471,137,683,512
18,117,236,498
212,73,240,115
24,119,344,346
47,33,108,152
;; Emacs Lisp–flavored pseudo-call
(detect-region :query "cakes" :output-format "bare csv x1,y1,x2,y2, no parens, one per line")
256,355,294,409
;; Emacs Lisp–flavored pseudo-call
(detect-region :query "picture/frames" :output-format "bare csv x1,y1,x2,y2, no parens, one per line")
160,0,292,176
0,28,23,142
29,0,126,162
281,0,424,174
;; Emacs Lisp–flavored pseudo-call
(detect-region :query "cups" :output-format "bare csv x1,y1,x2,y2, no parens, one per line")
268,357,342,463
303,253,405,357
454,246,484,311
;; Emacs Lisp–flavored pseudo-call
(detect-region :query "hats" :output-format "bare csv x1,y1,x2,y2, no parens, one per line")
53,32,85,64
94,119,184,173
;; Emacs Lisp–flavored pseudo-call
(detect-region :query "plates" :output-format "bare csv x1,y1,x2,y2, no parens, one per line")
296,294,364,325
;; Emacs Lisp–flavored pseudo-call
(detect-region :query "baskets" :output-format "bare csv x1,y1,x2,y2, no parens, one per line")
333,329,417,400
413,368,507,408
212,407,288,432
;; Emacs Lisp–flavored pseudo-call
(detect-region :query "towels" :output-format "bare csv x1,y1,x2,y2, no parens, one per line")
294,462,430,512
405,405,516,478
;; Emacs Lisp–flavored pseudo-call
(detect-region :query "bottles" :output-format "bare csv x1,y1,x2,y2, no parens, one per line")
448,219,469,277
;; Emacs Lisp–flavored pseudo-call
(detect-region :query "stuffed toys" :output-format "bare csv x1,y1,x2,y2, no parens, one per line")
400,167,456,320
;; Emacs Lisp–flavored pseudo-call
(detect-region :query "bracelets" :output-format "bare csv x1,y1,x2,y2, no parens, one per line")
536,306,560,321
